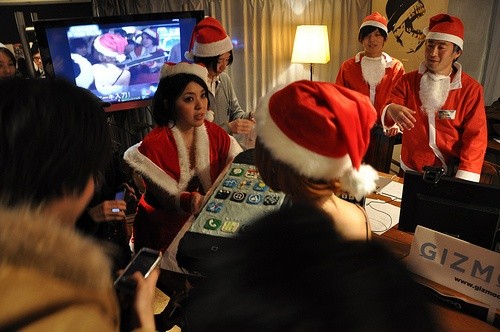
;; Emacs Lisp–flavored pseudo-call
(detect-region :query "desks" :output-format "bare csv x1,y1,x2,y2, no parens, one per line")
365,172,415,251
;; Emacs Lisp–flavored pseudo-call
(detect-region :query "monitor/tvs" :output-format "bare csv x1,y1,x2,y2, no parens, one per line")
398,171,500,253
33,9,204,112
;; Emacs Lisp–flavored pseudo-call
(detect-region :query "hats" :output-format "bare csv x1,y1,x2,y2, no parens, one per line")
135,30,142,37
255,80,378,200
184,17,233,61
358,12,388,35
158,62,208,83
143,28,157,37
423,14,465,50
94,33,128,63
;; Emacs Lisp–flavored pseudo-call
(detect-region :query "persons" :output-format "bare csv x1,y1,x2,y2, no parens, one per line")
0,47,17,80
336,12,406,174
255,80,378,242
381,13,488,183
0,80,160,332
75,169,138,272
29,43,46,78
123,62,244,286
68,25,182,102
185,17,258,138
182,206,442,332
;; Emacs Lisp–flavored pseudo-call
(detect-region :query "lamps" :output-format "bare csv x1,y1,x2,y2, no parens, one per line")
291,25,330,80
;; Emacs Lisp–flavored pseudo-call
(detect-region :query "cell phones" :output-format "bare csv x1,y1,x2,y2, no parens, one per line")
112,185,127,212
176,148,291,278
114,248,162,296
